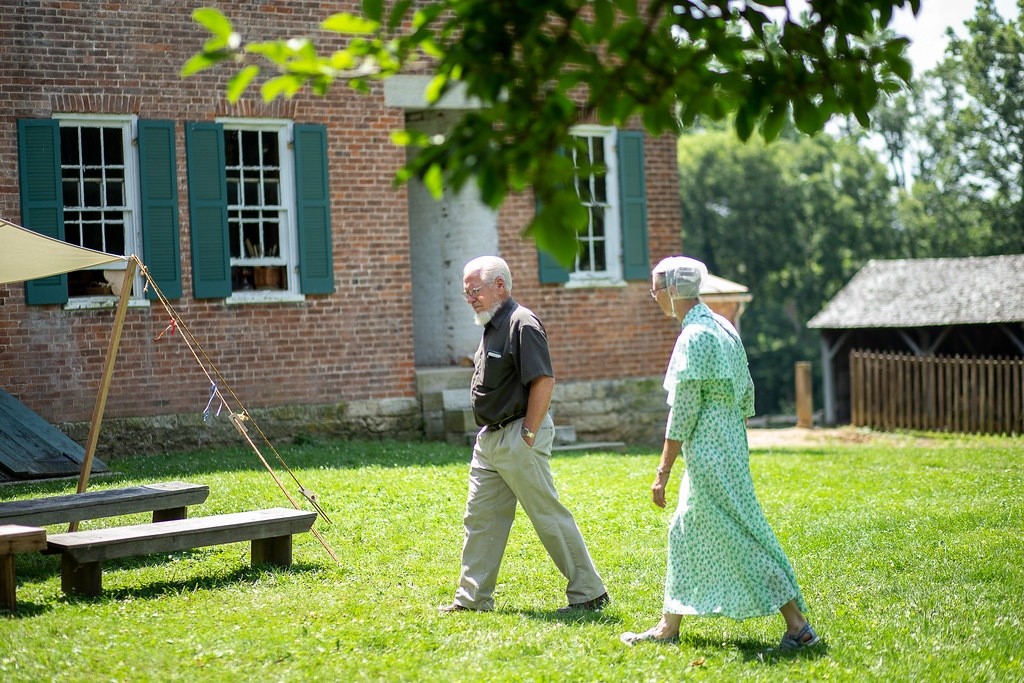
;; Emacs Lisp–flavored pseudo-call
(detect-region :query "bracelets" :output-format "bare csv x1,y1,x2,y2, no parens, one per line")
656,467,670,474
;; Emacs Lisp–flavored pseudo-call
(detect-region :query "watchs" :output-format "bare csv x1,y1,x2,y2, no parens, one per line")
519,428,536,440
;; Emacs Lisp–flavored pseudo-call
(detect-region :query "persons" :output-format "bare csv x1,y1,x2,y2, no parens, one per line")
438,256,610,611
622,256,833,659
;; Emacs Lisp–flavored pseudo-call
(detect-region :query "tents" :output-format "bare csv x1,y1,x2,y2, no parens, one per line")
0,214,136,539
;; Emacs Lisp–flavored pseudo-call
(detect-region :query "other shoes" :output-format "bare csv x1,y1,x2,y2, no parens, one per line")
436,603,494,615
557,591,609,616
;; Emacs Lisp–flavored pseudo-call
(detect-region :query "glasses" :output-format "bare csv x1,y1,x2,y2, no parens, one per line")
462,280,493,300
648,287,668,299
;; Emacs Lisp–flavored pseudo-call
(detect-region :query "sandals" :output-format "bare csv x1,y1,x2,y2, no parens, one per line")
620,627,679,650
767,621,821,655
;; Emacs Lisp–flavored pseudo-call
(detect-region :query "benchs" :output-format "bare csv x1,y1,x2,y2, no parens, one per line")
39,507,318,598
0,521,48,616
1,480,211,523
